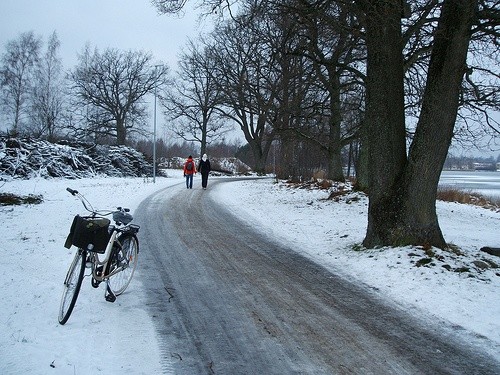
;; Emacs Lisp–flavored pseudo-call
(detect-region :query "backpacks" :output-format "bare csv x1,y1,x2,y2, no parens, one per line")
186,162,193,170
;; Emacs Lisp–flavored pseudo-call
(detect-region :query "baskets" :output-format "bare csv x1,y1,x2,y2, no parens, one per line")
70,215,114,254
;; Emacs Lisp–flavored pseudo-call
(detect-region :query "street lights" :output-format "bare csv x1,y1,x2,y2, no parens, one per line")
150,63,164,182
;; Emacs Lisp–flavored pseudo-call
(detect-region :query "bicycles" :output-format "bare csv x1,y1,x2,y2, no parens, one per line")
58,185,140,325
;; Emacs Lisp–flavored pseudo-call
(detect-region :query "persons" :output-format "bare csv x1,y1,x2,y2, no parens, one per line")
183,155,196,189
198,154,210,190
